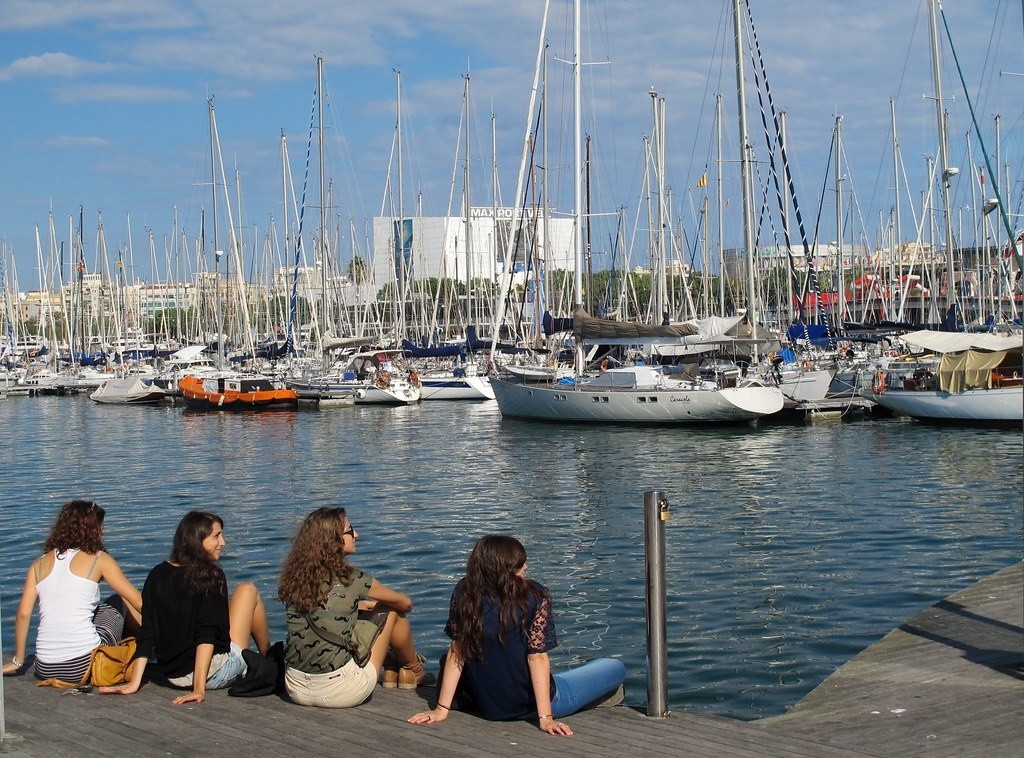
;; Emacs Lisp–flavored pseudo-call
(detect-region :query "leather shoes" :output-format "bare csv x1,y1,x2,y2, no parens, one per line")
382,661,436,690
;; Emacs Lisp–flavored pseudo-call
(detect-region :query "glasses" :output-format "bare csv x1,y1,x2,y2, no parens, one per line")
342,525,354,538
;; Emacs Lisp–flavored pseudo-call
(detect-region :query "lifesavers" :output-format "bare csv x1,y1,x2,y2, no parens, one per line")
600,359,608,372
376,377,388,390
871,372,885,394
801,359,810,372
839,347,848,358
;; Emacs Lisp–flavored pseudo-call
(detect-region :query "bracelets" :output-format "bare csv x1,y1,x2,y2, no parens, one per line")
539,715,552,720
12,656,23,667
437,702,451,711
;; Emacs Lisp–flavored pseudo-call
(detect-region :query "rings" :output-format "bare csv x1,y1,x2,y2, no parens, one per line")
427,716,430,721
557,723,563,727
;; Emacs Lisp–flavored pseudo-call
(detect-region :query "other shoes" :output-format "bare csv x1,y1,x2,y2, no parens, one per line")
584,684,626,707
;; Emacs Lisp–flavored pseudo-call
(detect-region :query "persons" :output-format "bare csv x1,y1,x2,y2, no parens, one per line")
407,533,626,736
278,506,437,708
99,510,287,705
2,500,143,683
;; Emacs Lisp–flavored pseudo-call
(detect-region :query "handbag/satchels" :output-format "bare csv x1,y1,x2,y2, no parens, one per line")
229,641,287,698
347,609,388,668
90,637,137,689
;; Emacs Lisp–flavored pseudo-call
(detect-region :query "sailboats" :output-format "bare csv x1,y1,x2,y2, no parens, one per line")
0,1,1023,420
